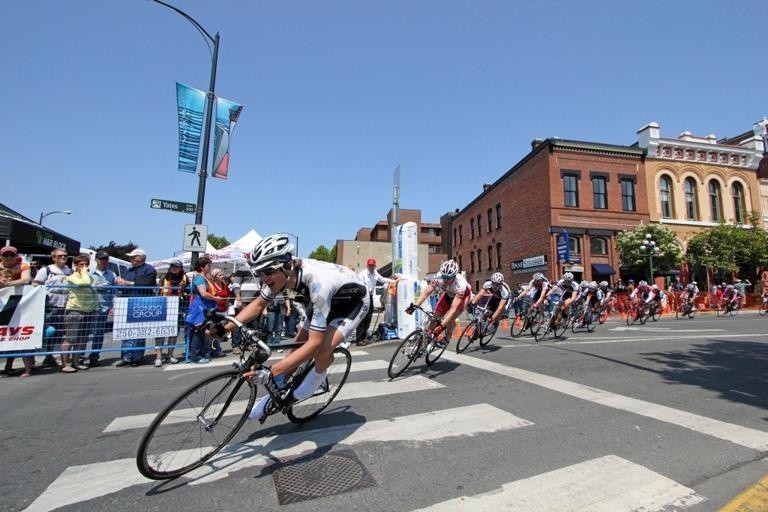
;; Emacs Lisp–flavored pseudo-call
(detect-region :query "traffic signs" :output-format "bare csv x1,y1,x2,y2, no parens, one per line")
151,198,195,213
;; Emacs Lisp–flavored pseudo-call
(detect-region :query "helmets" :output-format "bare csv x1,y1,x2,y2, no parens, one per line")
248,234,294,275
563,272,609,289
490,272,505,284
639,280,658,290
533,272,544,280
440,259,458,278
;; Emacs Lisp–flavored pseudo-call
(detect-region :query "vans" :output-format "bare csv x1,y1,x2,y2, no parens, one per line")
29,247,134,322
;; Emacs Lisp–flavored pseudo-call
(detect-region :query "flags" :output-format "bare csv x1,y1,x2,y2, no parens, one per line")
173,78,207,173
211,92,242,181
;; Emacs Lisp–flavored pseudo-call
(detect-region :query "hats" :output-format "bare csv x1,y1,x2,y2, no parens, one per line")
126,249,146,260
210,269,221,280
0,246,17,254
170,259,183,266
95,251,109,259
367,259,377,266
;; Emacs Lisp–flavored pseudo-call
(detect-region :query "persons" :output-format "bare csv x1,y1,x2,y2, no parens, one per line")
405,260,767,361
0,237,399,379
202,229,374,423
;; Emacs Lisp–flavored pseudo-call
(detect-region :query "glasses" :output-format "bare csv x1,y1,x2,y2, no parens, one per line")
254,269,278,275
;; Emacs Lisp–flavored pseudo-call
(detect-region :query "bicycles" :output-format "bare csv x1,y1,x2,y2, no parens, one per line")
675,300,698,321
626,295,663,327
135,307,354,481
758,300,768,318
456,304,499,354
716,303,740,318
508,298,613,342
386,302,453,380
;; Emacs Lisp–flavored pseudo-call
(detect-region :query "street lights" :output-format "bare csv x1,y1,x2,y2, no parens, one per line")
38,210,71,227
276,230,299,257
148,0,221,289
640,233,659,285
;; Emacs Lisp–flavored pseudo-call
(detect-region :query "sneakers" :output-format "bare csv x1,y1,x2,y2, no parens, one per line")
248,394,275,421
6,358,98,377
116,347,242,367
293,368,327,401
437,336,451,347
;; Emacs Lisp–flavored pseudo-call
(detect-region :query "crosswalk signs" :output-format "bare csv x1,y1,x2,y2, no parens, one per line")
184,223,206,251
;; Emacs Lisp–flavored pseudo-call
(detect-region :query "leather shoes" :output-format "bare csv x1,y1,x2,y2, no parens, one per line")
356,339,372,345
